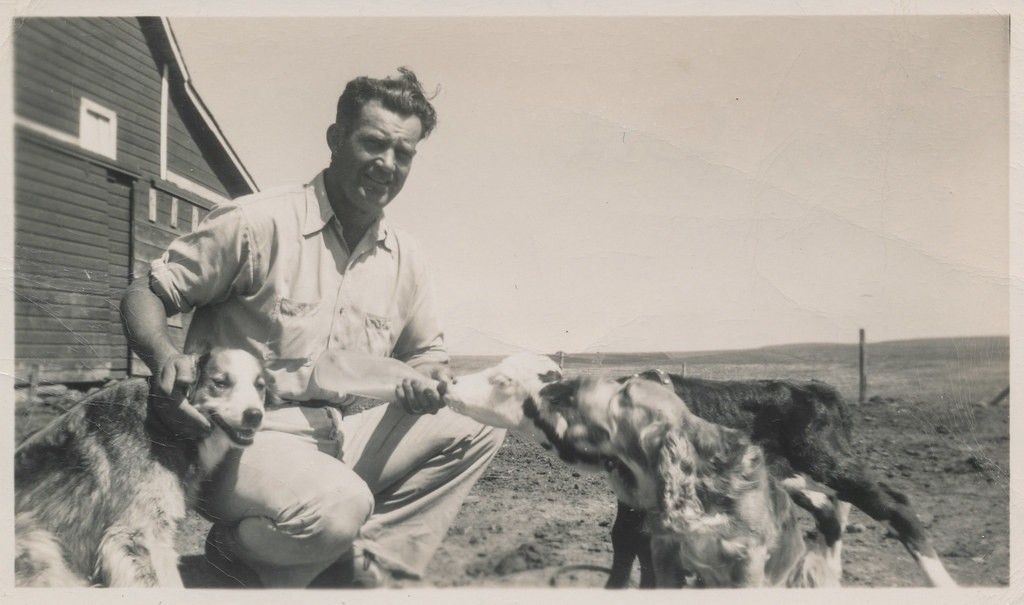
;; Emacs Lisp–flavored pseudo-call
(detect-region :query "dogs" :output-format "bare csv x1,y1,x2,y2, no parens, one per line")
15,346,269,590
441,348,962,590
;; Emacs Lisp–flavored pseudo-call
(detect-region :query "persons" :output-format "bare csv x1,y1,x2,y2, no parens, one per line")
119,73,509,591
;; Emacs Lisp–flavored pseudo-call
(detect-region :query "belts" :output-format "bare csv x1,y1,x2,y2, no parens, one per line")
267,399,343,410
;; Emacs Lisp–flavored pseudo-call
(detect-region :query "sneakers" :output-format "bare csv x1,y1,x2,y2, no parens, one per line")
205,523,261,588
318,547,395,588
